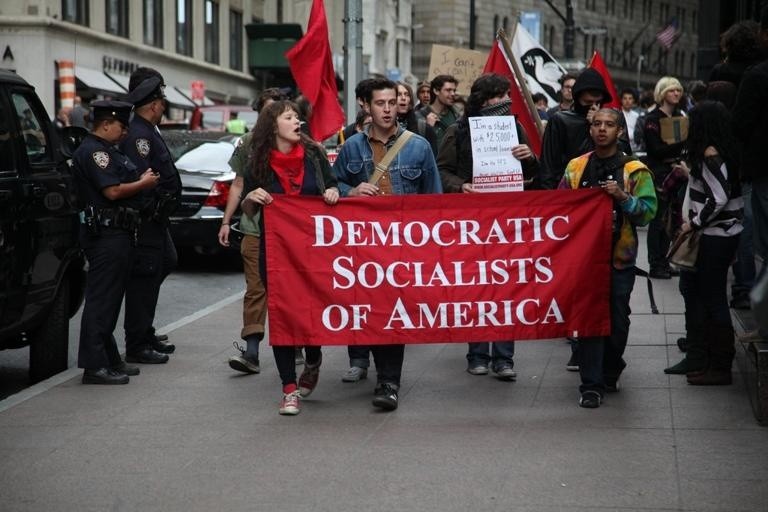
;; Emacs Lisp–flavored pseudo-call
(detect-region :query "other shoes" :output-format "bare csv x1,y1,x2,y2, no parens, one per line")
566,353,579,370
581,392,599,407
467,364,489,374
599,377,618,396
493,365,516,376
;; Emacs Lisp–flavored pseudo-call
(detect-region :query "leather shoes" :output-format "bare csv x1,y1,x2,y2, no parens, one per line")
82,336,175,384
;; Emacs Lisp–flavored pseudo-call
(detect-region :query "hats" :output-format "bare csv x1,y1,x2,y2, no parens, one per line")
129,78,166,108
654,77,682,103
89,101,133,128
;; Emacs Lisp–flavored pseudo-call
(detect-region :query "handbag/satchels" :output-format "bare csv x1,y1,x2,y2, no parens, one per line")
667,229,702,270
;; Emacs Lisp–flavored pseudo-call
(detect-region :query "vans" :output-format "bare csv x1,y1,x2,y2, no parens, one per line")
1,69,86,378
188,104,260,132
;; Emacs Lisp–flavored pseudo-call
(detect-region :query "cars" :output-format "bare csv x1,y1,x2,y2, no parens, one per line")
156,128,244,272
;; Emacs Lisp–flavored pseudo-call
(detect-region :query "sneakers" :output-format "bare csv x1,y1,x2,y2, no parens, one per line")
342,365,367,381
649,263,679,278
371,383,399,408
664,298,764,385
279,389,302,415
299,352,323,397
296,349,307,365
229,342,259,374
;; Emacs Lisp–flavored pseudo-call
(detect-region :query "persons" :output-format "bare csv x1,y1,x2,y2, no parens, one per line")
22,95,94,132
219,20,768,415
71,99,160,384
120,77,174,364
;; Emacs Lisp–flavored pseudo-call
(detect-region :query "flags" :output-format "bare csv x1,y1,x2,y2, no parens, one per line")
285,1,344,141
